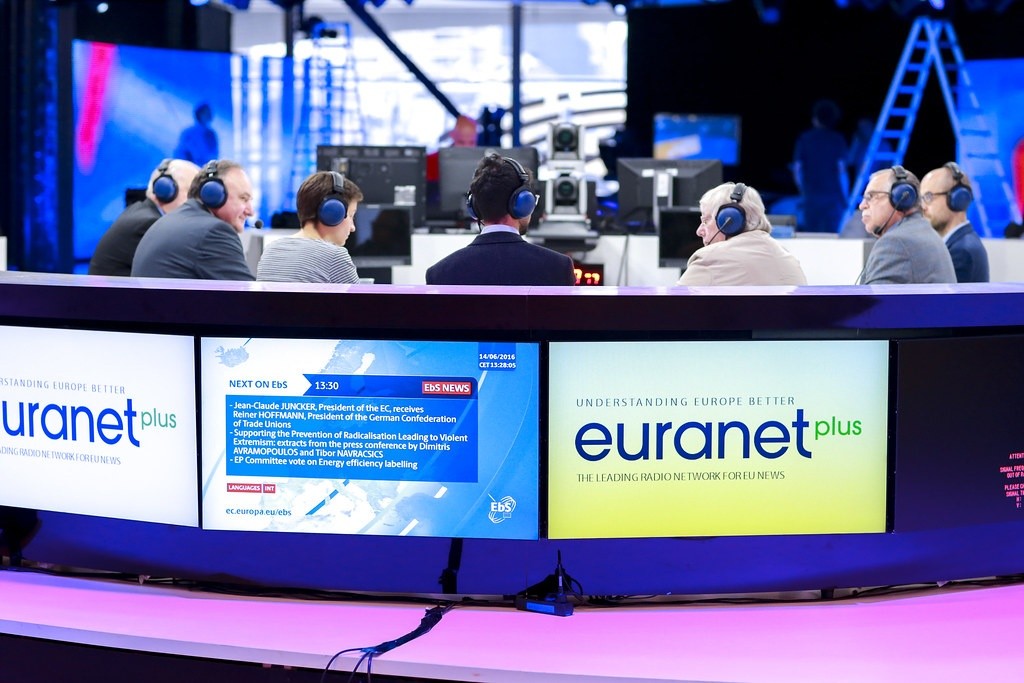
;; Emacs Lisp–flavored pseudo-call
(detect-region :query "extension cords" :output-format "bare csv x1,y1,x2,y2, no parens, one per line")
515,596,574,617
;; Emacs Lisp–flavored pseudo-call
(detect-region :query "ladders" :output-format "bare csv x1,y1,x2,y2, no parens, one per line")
283,53,369,219
837,16,1021,239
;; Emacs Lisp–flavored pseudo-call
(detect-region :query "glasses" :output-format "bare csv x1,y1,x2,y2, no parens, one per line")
918,192,947,204
862,191,890,202
535,195,540,205
700,216,712,228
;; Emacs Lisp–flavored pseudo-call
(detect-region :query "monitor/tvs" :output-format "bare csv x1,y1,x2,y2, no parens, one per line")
614,157,723,220
438,147,538,214
343,204,412,266
651,112,744,167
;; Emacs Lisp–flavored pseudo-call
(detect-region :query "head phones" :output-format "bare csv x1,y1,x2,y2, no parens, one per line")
152,158,179,203
944,162,973,212
716,183,748,236
199,159,228,208
317,171,347,226
888,165,918,213
465,158,536,221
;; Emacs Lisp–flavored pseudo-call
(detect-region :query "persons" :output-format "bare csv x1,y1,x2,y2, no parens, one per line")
859,165,958,285
256,170,364,284
919,162,990,283
793,99,892,233
425,115,477,205
674,181,808,286
130,159,257,281
425,152,577,286
172,103,219,168
87,158,203,277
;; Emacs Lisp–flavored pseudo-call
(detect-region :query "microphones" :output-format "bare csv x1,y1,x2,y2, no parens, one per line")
250,221,263,229
873,198,903,236
